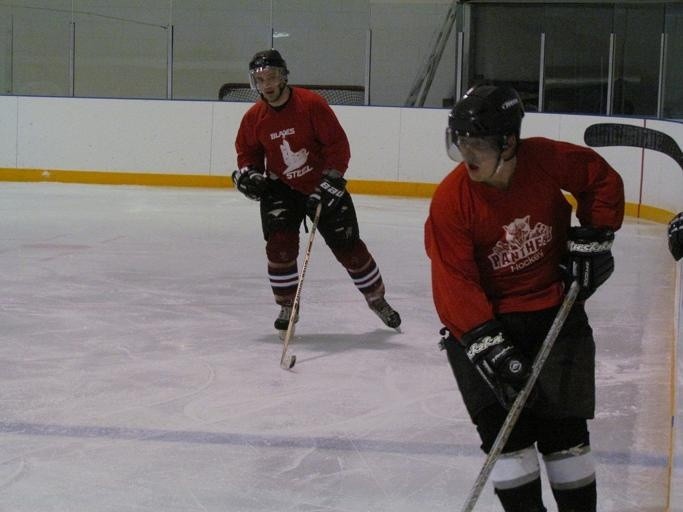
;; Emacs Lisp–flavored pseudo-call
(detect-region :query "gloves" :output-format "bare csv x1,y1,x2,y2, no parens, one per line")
551,223,617,303
458,318,550,418
229,166,281,210
309,173,350,220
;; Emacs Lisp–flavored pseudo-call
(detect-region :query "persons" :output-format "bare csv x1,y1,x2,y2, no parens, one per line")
423,81,625,512
667,211,683,262
232,50,402,328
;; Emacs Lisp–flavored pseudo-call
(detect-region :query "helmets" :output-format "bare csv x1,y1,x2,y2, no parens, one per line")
443,79,526,164
247,48,290,91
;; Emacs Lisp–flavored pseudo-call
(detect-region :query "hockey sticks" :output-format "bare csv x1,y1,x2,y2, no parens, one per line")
280,204,322,370
584,123,683,170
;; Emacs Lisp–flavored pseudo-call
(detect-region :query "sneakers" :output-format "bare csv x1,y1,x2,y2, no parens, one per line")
368,296,401,329
273,300,300,330
